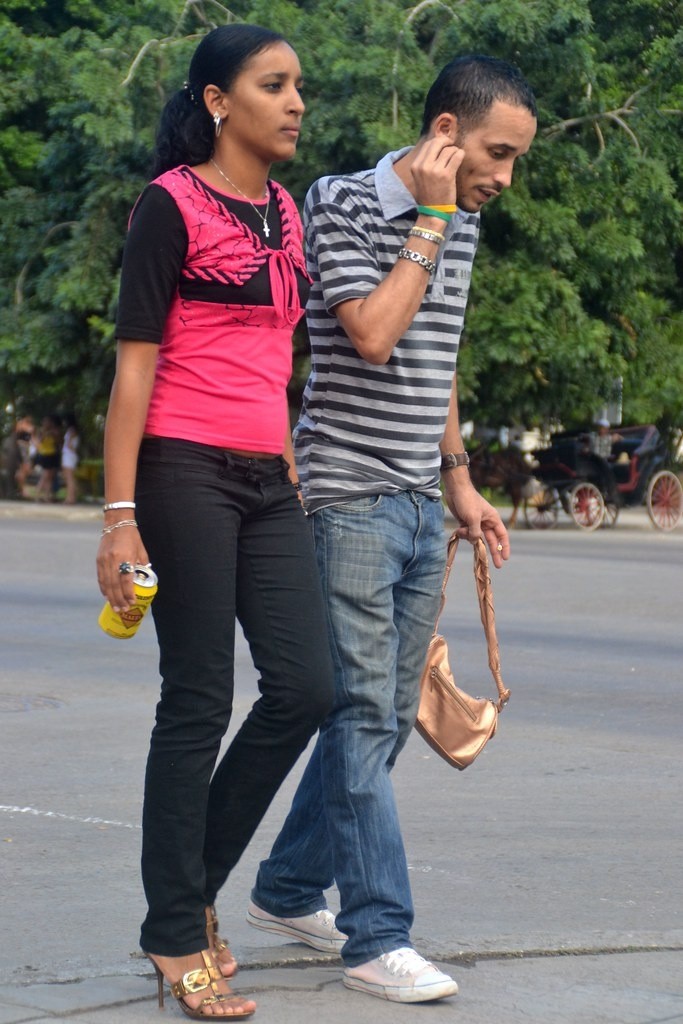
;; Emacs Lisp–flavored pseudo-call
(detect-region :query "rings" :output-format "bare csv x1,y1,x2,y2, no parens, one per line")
118,563,133,574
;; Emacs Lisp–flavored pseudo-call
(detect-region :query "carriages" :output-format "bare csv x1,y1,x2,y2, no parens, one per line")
457,422,683,532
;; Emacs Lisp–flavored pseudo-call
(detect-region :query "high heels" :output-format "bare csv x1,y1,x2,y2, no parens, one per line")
146,950,253,1019
206,905,238,980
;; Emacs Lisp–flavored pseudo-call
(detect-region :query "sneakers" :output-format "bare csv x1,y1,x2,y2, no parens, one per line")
342,948,459,1002
245,900,349,953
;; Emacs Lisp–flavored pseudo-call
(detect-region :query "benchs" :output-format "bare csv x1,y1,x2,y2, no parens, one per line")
612,439,642,484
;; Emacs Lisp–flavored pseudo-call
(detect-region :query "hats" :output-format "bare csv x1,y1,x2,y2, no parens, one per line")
597,419,610,428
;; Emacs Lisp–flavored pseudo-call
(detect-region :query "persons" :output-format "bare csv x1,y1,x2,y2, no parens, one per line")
0,414,64,501
247,54,541,1003
583,419,621,462
61,425,83,503
96,23,344,1023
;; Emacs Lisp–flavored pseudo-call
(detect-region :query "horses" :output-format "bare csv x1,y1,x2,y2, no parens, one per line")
468,444,531,528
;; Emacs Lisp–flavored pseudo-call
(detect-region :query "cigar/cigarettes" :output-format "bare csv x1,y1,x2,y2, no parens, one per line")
498,544,502,551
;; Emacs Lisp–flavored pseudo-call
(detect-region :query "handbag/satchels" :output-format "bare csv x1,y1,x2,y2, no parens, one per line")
414,632,498,772
17,433,31,441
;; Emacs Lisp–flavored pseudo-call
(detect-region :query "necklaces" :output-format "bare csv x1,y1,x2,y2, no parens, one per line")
210,158,270,238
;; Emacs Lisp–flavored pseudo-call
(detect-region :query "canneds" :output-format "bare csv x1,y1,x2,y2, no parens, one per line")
98,565,158,639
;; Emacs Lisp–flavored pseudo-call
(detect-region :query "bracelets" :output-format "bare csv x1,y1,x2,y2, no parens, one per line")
407,226,445,244
398,248,435,275
417,205,457,222
102,502,135,511
101,520,138,537
441,452,470,471
293,482,302,491
